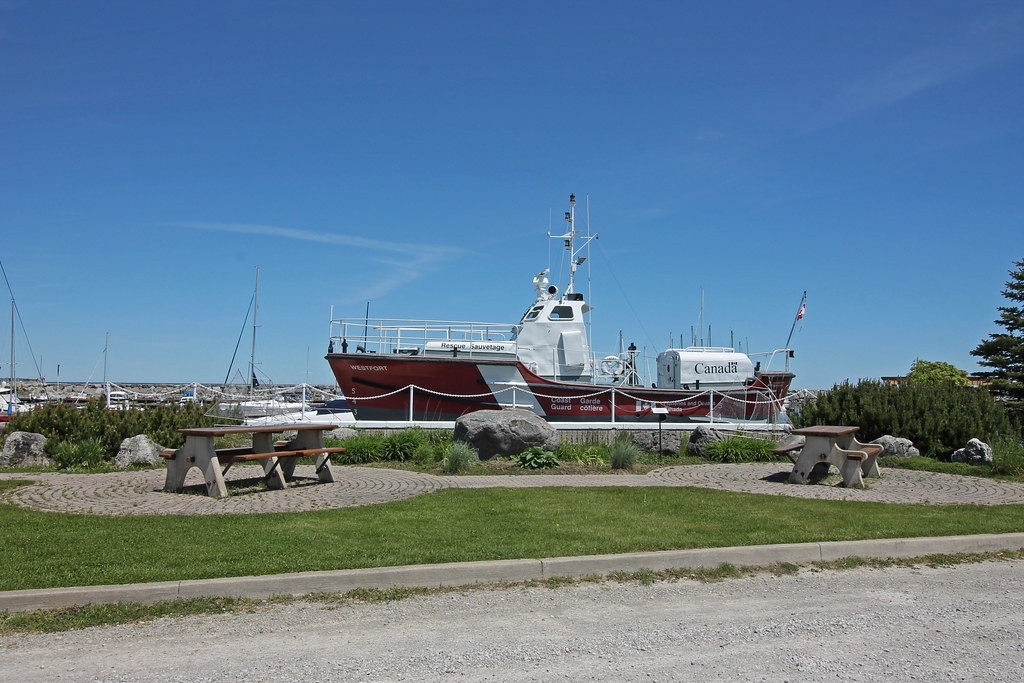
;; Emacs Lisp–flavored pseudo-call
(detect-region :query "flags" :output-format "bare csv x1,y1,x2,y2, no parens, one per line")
797,301,806,320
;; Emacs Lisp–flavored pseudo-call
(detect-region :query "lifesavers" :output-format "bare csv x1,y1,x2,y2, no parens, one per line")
600,355,625,377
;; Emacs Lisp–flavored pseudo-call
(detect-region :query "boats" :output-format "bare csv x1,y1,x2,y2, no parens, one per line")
0,247,355,430
325,191,809,425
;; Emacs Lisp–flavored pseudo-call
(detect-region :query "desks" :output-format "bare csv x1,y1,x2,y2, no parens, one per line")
788,425,881,489
161,424,340,498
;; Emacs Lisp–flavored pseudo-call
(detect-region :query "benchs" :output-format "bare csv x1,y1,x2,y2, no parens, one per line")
222,447,346,481
770,443,804,464
159,444,286,460
846,444,884,468
